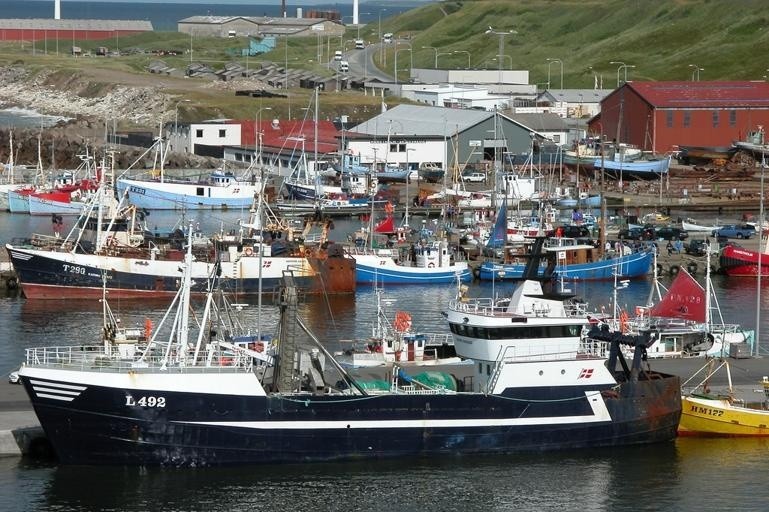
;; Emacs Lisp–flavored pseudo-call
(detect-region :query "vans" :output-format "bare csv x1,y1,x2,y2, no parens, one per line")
334,51,343,60
340,61,349,71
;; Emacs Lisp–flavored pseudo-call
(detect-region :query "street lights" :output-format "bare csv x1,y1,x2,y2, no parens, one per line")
485,26,518,70
422,46,452,68
546,58,563,89
610,62,636,87
454,51,470,68
689,65,704,81
394,40,413,84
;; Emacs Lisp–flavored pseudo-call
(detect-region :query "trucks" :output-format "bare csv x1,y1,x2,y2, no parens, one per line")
228,30,236,40
356,40,364,49
384,32,393,43
